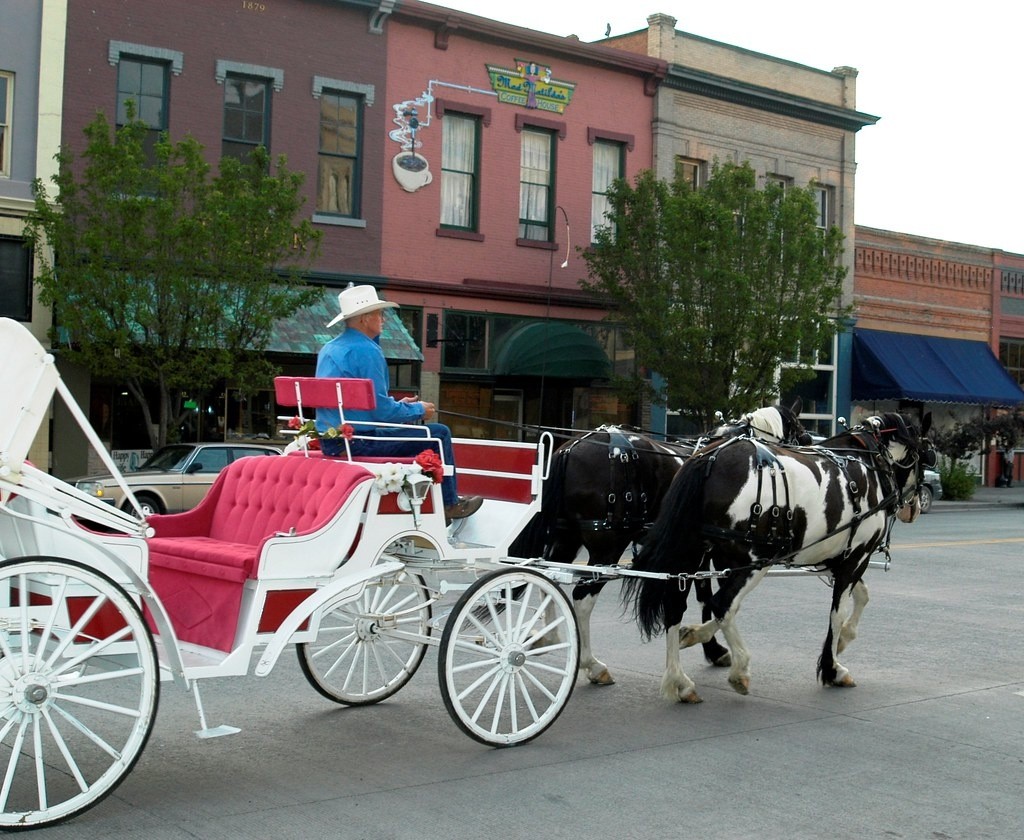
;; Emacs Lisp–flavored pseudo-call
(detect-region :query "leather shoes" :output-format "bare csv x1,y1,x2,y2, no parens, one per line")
445,492,485,523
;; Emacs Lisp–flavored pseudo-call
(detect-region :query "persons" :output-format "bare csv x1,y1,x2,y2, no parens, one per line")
90,397,113,453
314,284,484,527
996,439,1015,488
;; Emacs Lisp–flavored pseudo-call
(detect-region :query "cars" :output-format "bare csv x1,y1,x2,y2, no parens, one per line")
920,469,943,514
52,442,287,533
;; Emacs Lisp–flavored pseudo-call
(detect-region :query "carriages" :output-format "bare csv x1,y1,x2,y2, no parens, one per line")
0,317,940,833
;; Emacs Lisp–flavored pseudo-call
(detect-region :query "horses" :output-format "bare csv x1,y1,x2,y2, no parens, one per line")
454,393,940,706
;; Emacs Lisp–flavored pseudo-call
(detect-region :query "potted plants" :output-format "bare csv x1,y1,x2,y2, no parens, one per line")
939,451,978,502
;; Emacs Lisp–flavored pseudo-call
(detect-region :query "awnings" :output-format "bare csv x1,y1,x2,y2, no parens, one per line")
486,317,618,379
54,266,426,362
851,326,1024,411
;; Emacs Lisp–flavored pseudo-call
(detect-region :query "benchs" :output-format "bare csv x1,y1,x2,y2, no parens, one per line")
0,316,455,683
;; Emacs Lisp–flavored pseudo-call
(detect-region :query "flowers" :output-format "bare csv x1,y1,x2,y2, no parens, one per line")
288,415,355,446
375,448,445,495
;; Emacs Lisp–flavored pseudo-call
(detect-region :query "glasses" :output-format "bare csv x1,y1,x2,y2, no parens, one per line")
375,309,385,319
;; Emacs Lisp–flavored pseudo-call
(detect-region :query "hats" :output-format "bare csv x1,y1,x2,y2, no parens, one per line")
324,285,402,330
